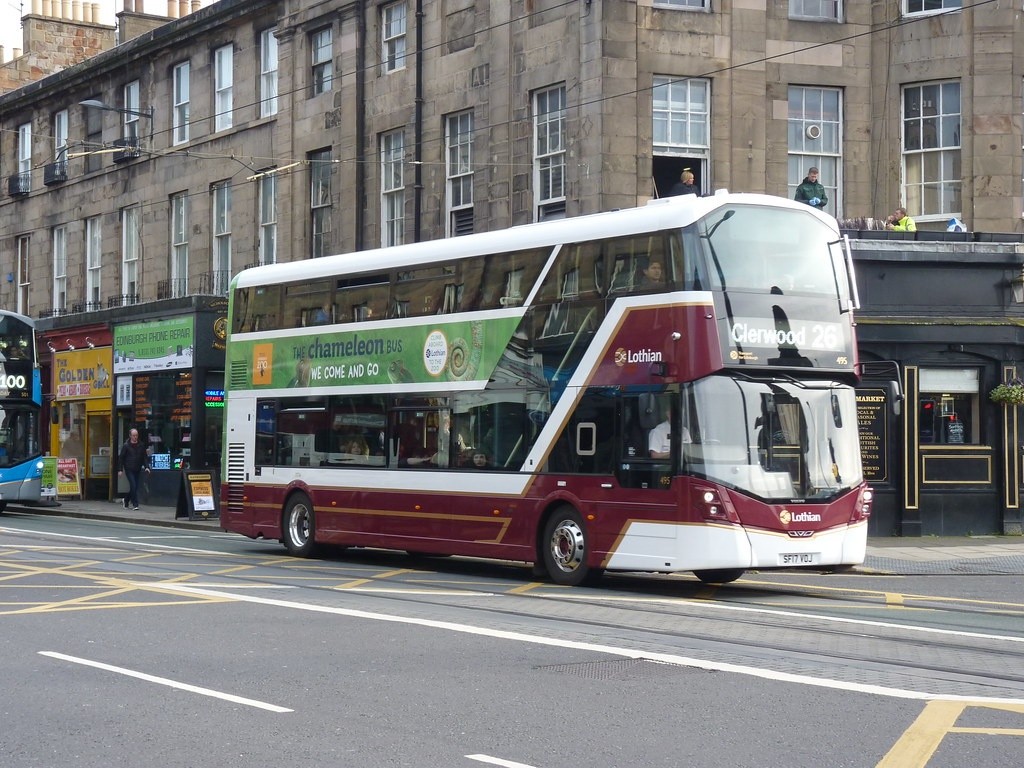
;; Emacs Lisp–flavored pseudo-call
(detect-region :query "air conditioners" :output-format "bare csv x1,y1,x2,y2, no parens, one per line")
112,138,140,163
7,174,30,197
43,161,67,186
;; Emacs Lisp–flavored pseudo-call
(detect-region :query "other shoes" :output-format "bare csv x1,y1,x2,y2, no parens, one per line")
122,498,129,510
133,507,139,510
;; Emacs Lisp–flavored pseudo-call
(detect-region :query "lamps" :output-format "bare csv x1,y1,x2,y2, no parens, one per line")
86,337,95,350
8,272,16,283
47,342,57,354
66,339,76,351
78,100,154,140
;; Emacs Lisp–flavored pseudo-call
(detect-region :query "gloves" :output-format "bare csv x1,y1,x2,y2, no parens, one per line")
809,200,816,205
814,197,821,204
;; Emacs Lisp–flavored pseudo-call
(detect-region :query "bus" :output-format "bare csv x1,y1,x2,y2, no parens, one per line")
213,190,907,587
0,308,60,518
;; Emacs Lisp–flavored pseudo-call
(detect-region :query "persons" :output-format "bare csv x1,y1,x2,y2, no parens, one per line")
390,415,466,469
6,346,32,360
646,404,693,468
117,428,151,511
467,448,492,467
669,170,701,197
315,298,330,321
344,439,369,465
794,166,828,211
886,207,917,231
639,259,667,290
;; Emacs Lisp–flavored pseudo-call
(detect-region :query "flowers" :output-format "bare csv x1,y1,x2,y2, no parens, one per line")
989,379,1024,405
